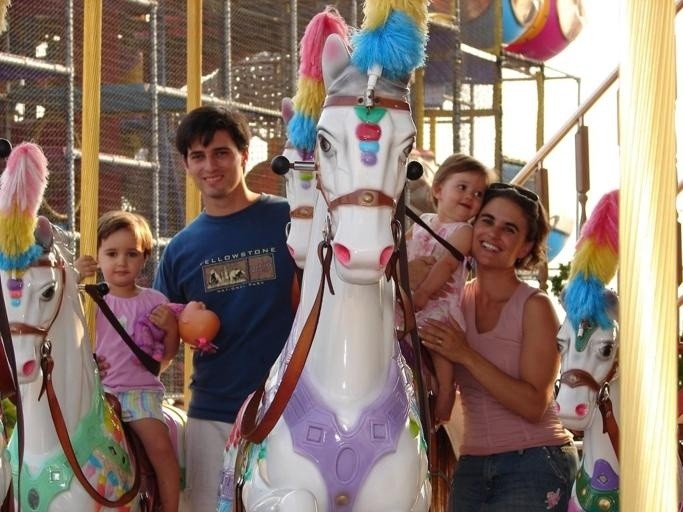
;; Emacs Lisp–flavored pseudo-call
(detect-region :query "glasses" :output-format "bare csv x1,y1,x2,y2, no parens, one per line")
490,183,539,201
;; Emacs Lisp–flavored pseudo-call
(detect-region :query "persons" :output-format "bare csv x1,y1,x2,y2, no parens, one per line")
408,183,579,512
75,211,180,512
406,154,488,422
131,300,220,369
96,106,303,512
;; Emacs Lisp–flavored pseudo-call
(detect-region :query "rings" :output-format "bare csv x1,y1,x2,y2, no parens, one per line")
437,339,442,345
